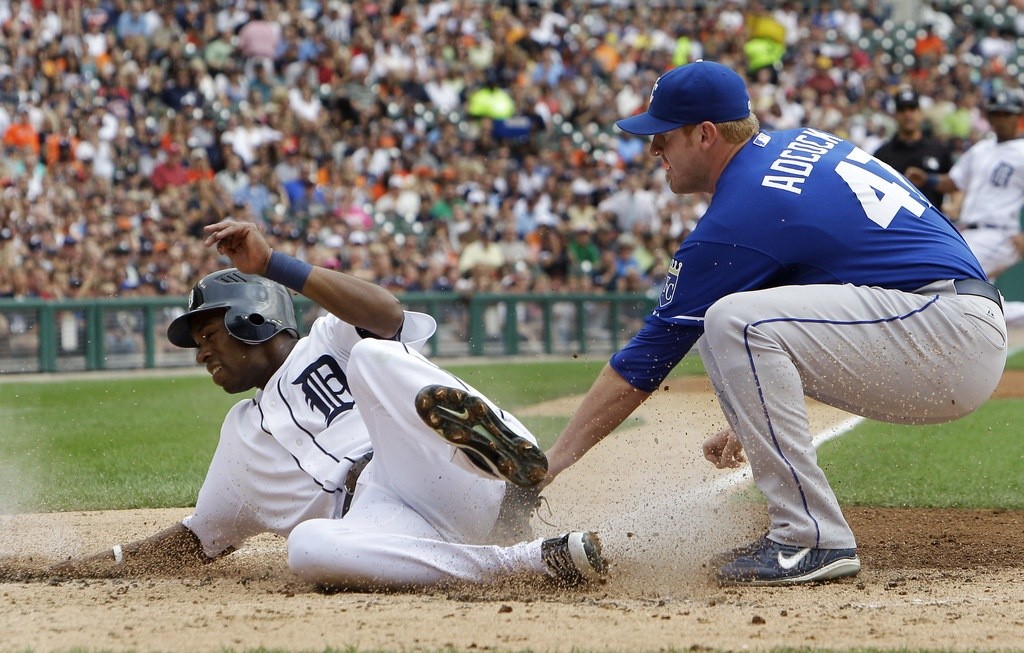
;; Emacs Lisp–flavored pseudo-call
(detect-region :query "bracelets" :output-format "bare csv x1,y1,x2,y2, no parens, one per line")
111,544,123,564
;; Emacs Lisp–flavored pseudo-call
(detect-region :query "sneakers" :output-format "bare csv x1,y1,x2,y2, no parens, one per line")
538,529,613,594
709,529,863,585
409,380,552,491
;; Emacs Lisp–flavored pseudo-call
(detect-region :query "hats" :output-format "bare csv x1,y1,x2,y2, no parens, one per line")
892,89,918,107
986,93,1022,116
616,60,755,140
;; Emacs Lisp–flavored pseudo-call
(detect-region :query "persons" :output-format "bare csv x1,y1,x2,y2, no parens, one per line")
509,57,1009,590
0,0,1024,360
34,218,609,594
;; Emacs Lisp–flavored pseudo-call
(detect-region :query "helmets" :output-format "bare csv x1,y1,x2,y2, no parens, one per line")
161,267,298,346
